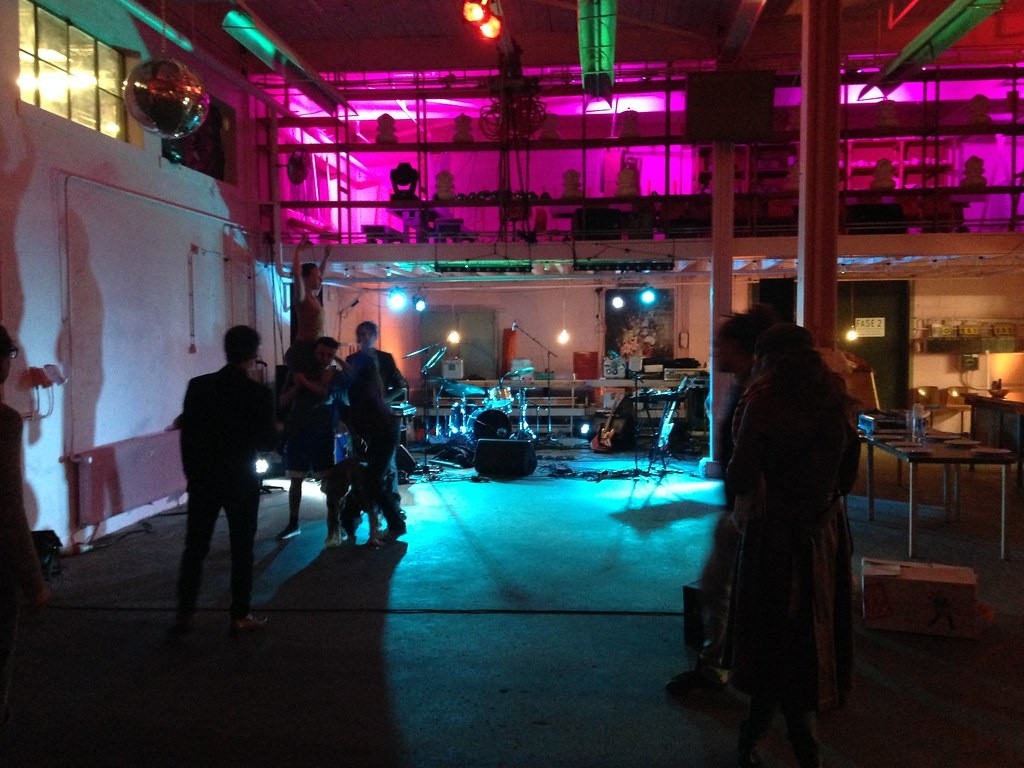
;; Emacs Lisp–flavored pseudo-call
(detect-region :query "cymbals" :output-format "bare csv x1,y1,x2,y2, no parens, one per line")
507,366,535,377
443,382,486,396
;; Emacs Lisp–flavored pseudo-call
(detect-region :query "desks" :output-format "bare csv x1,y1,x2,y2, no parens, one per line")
841,414,1014,556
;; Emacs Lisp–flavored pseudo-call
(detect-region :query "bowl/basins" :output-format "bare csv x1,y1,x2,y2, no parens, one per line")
988,390,1010,398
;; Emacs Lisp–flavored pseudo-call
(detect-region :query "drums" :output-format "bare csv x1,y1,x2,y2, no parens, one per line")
467,408,512,446
488,386,513,408
451,403,485,427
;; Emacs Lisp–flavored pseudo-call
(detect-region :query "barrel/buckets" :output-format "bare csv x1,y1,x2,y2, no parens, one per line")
572,351,598,379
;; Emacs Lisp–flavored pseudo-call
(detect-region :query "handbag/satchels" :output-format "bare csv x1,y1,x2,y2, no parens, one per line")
29,530,63,575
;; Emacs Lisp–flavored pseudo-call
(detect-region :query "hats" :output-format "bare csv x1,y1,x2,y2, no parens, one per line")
754,322,813,355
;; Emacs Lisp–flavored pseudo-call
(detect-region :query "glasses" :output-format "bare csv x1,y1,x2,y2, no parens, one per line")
8,345,18,359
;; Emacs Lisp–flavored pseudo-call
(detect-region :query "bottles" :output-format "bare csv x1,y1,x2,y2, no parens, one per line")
991,379,1002,389
911,401,926,440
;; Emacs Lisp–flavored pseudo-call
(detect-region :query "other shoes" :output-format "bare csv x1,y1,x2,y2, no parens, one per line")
176,611,201,627
277,524,302,539
383,525,405,540
368,537,380,547
231,616,273,634
665,668,728,697
737,736,761,768
341,536,355,546
325,535,341,547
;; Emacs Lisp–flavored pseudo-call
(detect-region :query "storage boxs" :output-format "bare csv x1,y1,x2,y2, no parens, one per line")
858,556,987,638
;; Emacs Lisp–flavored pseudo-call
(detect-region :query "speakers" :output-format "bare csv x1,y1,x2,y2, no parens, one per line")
472,438,537,481
396,444,416,478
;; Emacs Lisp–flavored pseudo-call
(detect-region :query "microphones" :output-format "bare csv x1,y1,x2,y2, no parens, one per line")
512,320,517,330
608,350,622,359
500,429,507,435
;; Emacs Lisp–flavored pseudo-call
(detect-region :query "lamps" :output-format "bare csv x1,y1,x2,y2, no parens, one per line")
446,295,461,345
221,1,358,119
558,290,569,345
411,288,427,312
857,0,1010,101
577,0,617,90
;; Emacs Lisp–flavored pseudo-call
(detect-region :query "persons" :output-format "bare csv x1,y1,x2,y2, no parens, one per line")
337,348,406,548
173,324,285,632
276,338,342,546
358,320,410,402
291,233,332,340
663,304,862,768
0,325,51,725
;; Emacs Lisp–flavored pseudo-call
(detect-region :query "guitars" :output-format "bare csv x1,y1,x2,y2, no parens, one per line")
657,375,688,448
589,391,622,453
